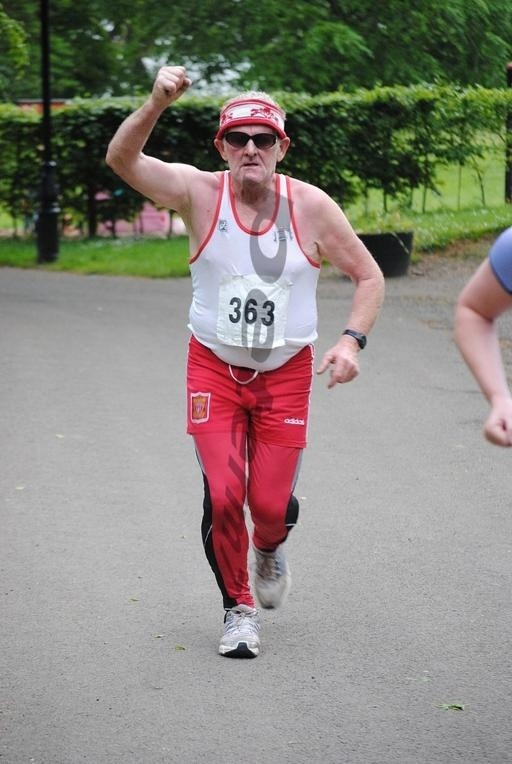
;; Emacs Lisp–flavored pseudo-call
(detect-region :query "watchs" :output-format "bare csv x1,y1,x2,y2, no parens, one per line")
342,329,367,350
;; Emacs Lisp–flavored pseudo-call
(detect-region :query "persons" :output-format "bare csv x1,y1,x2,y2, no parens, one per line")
105,66,384,659
453,224,511,446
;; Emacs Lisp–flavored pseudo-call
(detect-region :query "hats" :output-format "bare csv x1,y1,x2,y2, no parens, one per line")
214,92,290,143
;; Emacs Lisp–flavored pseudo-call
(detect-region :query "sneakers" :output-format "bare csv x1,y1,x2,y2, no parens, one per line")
249,544,292,610
217,600,262,658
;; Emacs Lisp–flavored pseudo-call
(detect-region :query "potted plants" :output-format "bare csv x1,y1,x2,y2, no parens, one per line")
353,215,415,279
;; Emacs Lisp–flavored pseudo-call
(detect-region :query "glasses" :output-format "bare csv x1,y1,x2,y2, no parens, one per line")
222,131,277,151
